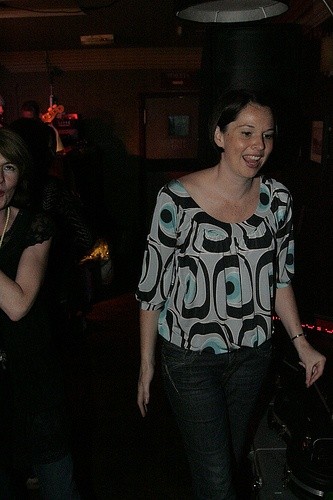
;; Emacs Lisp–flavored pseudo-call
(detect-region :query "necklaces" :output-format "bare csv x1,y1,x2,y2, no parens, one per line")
0,207,10,247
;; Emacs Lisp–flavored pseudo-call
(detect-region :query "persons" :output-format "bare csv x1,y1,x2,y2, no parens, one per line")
0,97,103,209
135,90,327,500
0,127,52,500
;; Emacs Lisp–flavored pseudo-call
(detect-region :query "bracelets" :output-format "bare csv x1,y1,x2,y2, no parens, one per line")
291,332,304,341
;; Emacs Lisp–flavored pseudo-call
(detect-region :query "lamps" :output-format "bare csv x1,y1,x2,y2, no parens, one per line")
173,0,289,25
